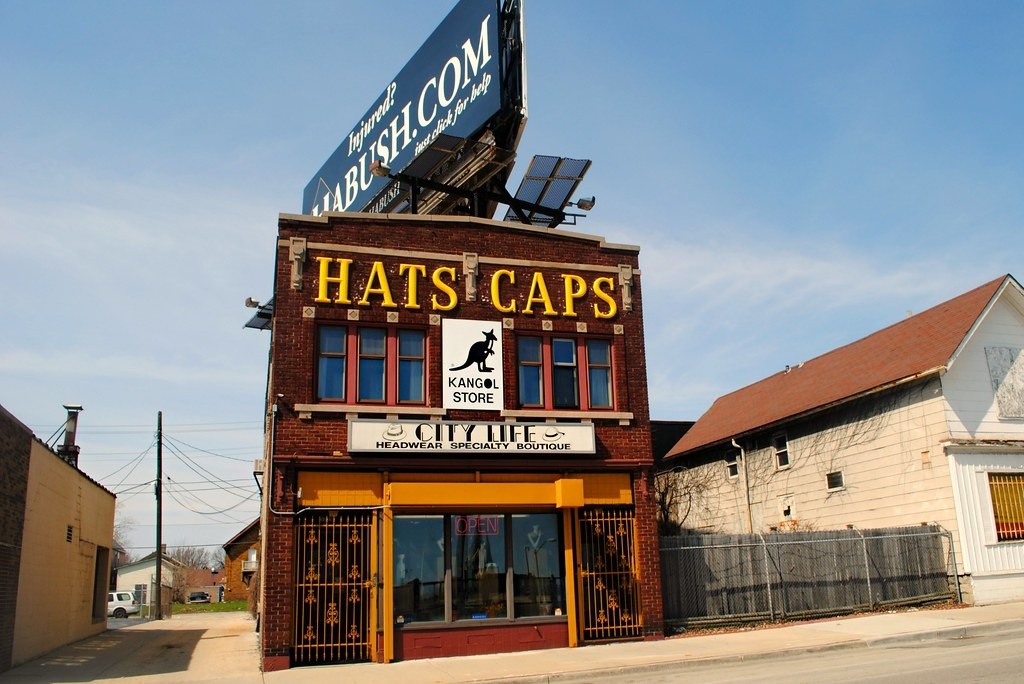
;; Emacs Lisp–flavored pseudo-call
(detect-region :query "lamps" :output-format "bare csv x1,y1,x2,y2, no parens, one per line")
245,297,262,309
369,161,395,181
567,196,595,210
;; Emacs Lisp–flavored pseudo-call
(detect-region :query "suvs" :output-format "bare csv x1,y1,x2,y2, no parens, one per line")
107,590,140,619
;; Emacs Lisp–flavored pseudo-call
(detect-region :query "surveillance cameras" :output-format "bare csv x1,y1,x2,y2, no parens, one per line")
278,394,285,398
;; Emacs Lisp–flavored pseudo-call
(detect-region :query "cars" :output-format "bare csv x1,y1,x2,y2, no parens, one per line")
188,591,213,604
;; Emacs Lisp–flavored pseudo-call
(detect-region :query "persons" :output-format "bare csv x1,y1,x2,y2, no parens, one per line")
527,520,556,605
437,531,452,556
